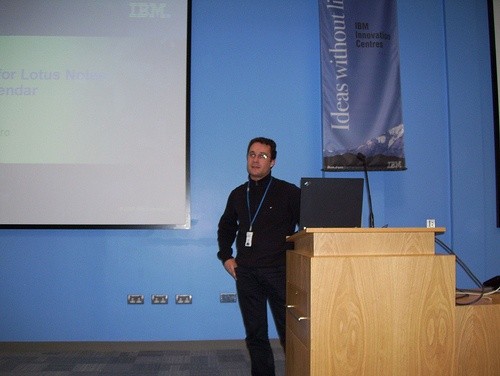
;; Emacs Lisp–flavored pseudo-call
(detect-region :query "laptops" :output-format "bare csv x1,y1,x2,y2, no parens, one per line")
290,178,364,235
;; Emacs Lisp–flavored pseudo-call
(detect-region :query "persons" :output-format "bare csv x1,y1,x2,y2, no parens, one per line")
217,137,301,376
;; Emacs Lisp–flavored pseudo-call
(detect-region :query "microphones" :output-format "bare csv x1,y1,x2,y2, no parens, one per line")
358,150,374,228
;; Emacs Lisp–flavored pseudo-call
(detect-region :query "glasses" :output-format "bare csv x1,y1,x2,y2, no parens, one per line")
248,151,272,160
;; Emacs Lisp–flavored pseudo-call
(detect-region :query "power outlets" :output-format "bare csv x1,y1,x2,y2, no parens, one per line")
176,295,192,303
220,293,237,303
152,295,168,304
128,295,144,304
